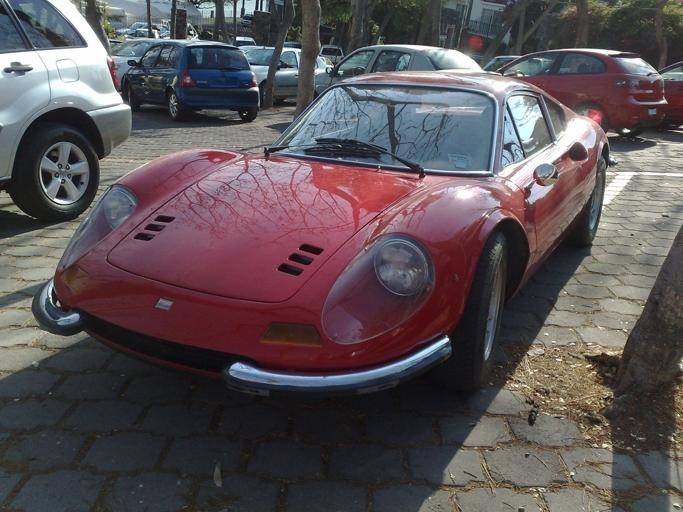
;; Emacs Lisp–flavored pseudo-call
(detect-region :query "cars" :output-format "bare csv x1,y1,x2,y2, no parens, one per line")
234,37,343,107
110,42,261,122
115,19,199,40
315,42,485,101
483,48,682,136
34,70,609,409
0,1,132,223
